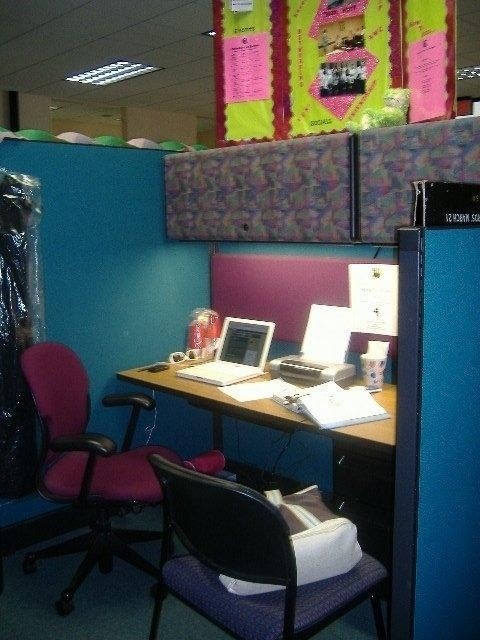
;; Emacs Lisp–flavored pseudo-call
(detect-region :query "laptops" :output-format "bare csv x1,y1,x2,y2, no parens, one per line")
177,316,276,387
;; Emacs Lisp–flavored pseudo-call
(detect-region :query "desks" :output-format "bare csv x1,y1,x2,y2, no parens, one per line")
115,353,397,543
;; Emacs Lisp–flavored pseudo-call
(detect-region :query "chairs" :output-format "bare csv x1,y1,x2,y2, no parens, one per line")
146,449,389,640
17,341,227,618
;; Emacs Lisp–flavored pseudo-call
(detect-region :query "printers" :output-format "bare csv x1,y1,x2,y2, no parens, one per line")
269,355,356,388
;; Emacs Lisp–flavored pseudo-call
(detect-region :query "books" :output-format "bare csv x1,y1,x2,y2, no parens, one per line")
272,380,346,416
296,384,392,432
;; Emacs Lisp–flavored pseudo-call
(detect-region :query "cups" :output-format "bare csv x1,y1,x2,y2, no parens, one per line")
361,354,388,392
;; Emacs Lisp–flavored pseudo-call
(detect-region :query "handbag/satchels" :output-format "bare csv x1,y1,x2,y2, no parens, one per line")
216,480,364,597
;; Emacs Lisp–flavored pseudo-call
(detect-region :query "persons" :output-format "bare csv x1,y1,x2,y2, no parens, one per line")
321,60,365,96
